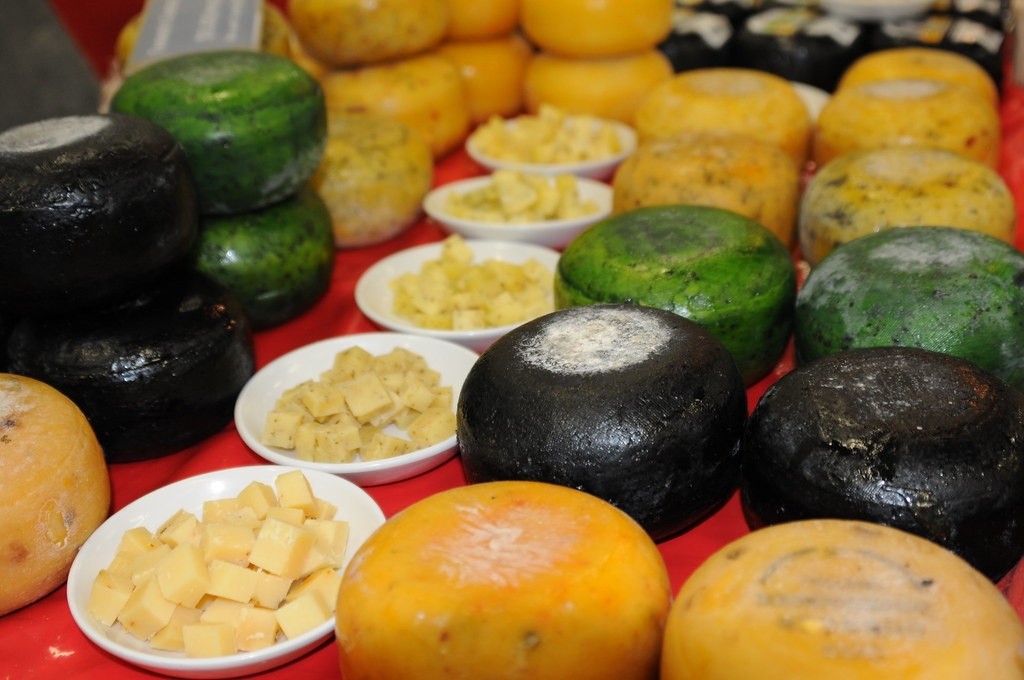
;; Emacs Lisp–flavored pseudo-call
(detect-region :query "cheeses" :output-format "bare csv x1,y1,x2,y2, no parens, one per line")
83,105,625,655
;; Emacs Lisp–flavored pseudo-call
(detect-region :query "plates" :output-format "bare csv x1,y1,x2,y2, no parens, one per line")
354,239,564,354
423,171,616,248
464,116,638,181
68,465,388,678
234,332,482,485
